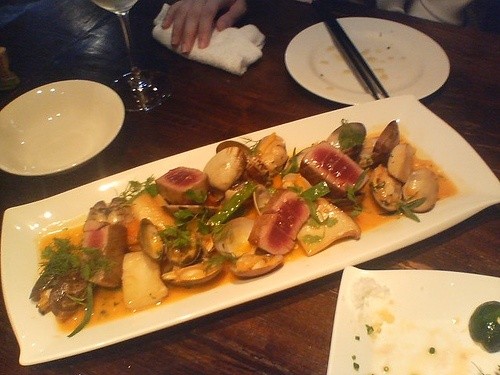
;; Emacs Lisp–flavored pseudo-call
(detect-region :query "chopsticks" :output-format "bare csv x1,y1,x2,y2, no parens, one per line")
311,0,390,100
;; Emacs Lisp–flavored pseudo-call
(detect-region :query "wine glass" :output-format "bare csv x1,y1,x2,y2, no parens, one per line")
90,0,173,115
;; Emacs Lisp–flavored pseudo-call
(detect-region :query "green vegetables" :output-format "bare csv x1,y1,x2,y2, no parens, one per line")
37,125,429,339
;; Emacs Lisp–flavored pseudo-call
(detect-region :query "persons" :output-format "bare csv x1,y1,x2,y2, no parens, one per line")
162,0,247,55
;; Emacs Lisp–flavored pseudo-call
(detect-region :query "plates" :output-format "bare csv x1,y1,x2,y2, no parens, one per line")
283,16,451,107
0,93,500,367
326,266,500,375
0,79,126,177
